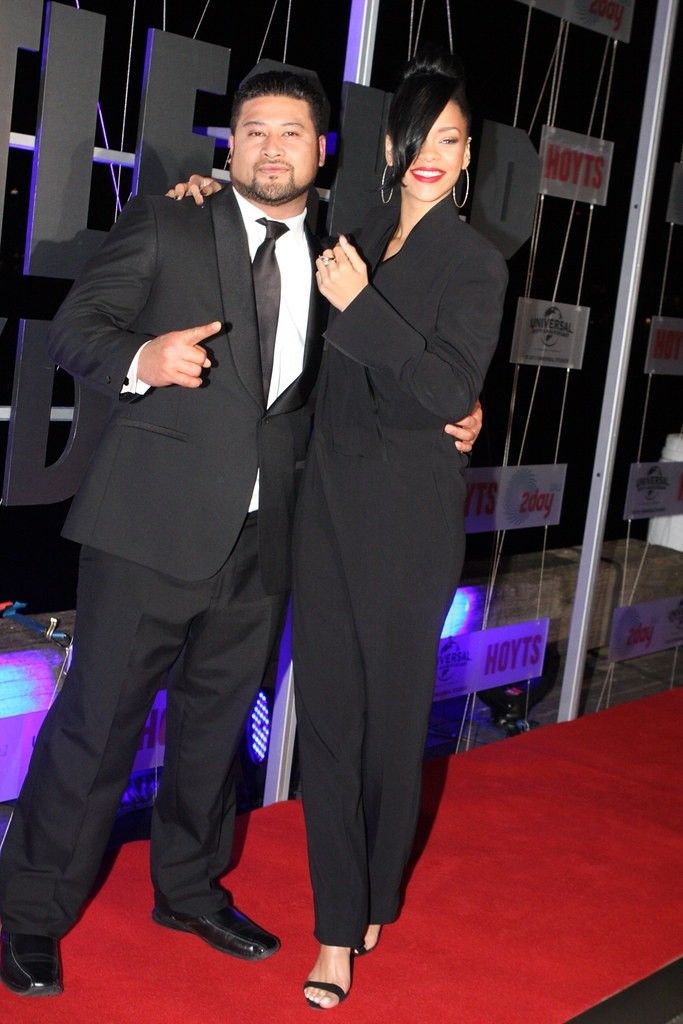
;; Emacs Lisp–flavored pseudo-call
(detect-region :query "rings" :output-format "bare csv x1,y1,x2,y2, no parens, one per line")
322,257,334,265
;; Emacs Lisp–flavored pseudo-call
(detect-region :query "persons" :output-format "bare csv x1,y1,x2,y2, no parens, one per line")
164,54,510,1010
0,71,483,996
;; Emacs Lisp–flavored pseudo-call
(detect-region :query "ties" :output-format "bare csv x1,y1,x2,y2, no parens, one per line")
247,216,289,403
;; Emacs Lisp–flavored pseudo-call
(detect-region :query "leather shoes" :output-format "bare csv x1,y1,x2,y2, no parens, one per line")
151,894,281,961
0,931,62,998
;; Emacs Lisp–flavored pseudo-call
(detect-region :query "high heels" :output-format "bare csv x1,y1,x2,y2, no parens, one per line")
302,921,383,1009
